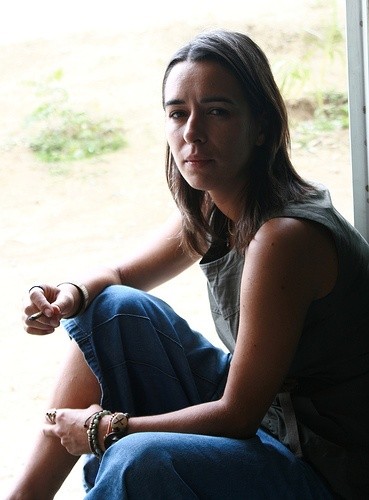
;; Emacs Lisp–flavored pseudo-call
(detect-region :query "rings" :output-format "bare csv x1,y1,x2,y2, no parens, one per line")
46,407,57,424
28,285,46,294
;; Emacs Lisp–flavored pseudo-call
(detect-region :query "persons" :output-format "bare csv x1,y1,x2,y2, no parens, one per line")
1,31,369,500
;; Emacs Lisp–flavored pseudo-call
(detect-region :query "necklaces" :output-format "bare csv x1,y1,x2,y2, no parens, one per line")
227,217,241,237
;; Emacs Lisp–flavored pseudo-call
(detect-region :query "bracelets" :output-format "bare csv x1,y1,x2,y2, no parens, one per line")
83,410,130,459
57,280,89,320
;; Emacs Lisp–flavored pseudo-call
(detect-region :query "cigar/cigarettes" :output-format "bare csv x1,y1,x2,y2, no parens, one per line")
27,310,44,321
